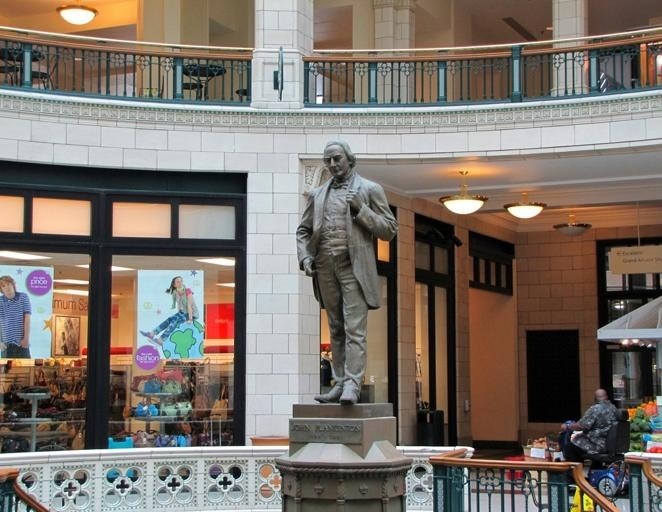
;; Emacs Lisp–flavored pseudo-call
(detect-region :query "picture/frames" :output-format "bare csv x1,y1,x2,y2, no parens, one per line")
55,316,80,356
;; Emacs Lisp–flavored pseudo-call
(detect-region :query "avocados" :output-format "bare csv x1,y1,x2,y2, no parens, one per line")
628,410,652,452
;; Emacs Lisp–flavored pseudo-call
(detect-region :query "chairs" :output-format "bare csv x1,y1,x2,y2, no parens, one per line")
183,83,203,100
32,55,59,90
0,66,19,86
236,89,248,96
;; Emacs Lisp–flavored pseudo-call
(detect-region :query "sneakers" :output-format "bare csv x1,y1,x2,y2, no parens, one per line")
140,331,154,339
151,338,163,346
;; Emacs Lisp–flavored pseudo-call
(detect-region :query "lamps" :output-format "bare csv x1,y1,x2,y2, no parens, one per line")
56,1,99,26
439,170,489,216
504,192,547,219
553,213,592,237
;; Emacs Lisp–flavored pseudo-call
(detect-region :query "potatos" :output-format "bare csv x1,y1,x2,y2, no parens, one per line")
532,438,546,448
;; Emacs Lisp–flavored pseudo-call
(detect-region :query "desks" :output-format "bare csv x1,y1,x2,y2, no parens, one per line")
0,48,45,87
183,64,226,100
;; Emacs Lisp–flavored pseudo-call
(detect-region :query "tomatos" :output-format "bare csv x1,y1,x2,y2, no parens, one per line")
650,447,662,452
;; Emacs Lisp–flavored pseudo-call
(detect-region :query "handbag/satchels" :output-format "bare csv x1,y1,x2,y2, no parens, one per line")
108,368,236,450
1,360,86,453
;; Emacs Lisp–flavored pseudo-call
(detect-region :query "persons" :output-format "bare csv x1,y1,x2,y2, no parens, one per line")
139,276,200,348
562,388,619,463
296,140,399,407
0,275,32,359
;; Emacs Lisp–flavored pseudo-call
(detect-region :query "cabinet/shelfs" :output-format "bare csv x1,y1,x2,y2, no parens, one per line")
0,365,131,453
134,357,234,447
